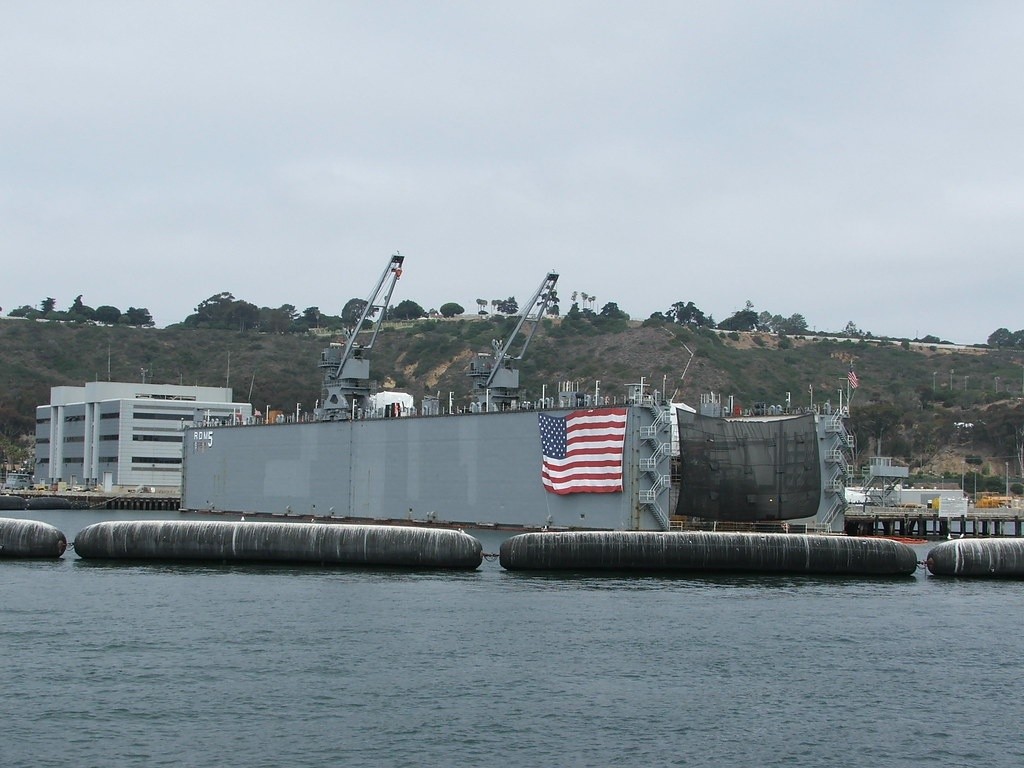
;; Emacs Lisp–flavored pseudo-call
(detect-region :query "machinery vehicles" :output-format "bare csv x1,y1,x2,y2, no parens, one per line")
317,248,406,421
462,267,560,412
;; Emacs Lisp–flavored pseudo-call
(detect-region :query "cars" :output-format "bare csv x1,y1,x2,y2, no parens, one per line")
30,483,156,494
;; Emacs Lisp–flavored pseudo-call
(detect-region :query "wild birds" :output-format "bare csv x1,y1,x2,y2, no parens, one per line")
240,516,246,522
947,532,965,540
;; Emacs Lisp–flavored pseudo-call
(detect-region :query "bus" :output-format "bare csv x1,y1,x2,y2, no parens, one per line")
4,472,35,489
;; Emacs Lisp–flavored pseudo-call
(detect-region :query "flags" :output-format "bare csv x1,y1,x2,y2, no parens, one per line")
538,408,629,495
